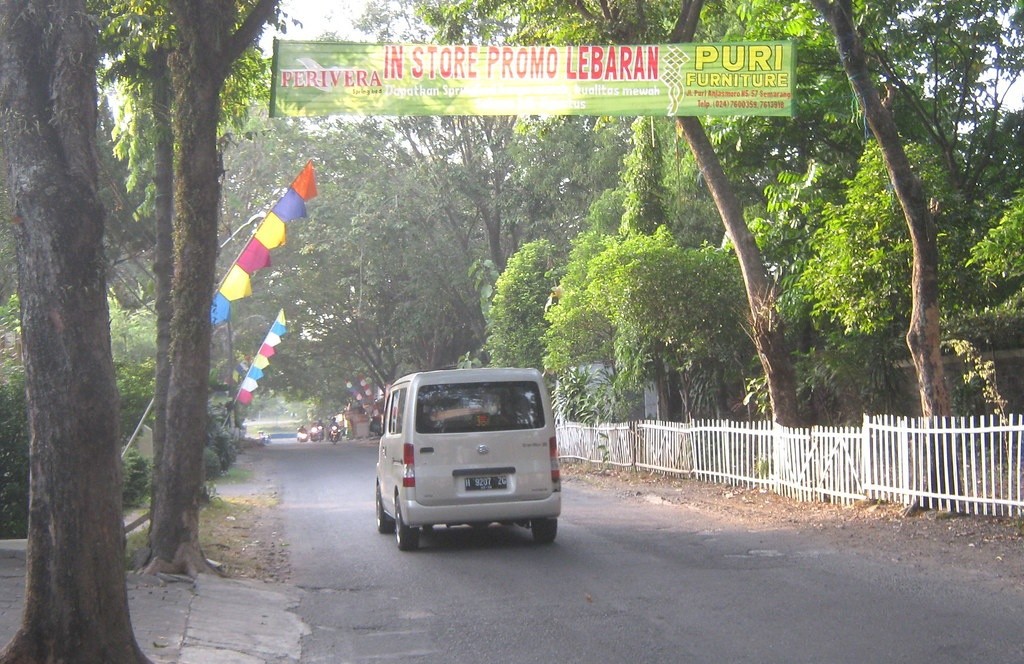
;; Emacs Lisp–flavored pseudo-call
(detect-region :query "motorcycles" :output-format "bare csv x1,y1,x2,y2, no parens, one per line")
328,426,341,444
297,431,308,443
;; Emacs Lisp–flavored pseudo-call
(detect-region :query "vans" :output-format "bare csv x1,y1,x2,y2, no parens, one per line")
373,366,562,550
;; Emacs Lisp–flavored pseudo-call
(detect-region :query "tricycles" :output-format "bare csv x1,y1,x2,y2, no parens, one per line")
311,426,325,443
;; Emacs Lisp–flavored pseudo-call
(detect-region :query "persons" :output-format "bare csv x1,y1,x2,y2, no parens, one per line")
292,414,345,446
